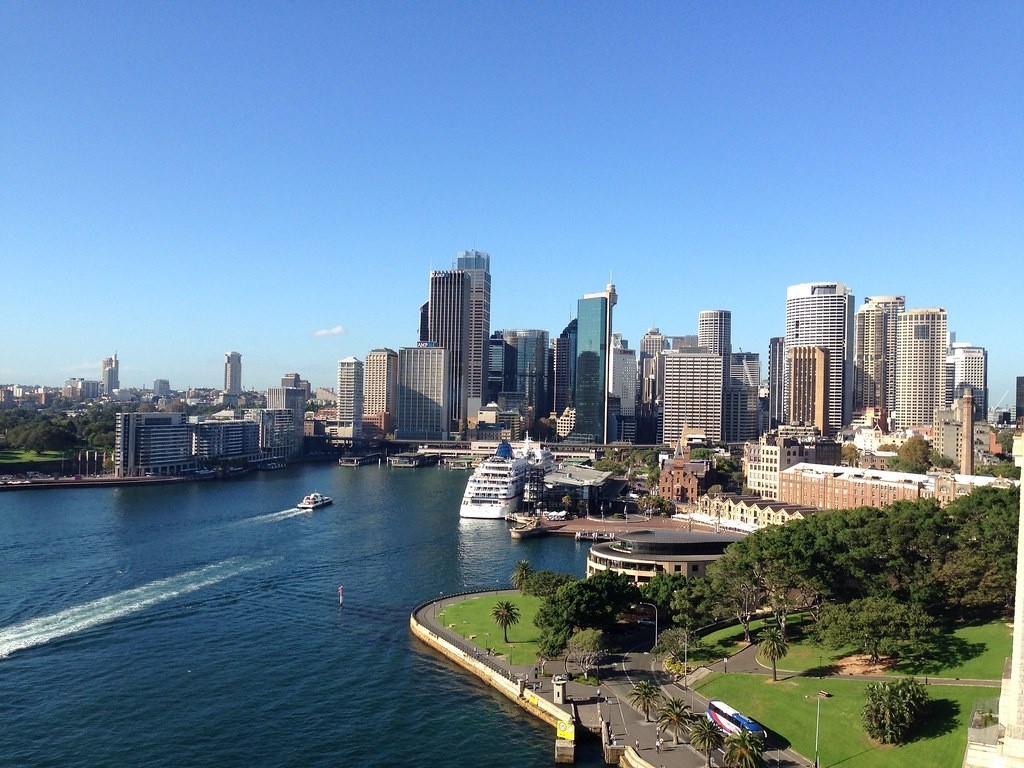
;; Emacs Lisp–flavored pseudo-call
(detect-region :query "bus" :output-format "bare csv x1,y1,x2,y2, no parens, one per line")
707,700,767,753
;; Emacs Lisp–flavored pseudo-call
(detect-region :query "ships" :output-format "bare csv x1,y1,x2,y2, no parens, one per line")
460,429,558,519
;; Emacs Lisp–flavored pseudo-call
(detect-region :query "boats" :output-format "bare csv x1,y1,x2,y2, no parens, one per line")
508,516,544,539
297,490,334,510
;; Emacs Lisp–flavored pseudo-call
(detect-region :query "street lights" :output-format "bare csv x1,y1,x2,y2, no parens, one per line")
631,602,659,649
